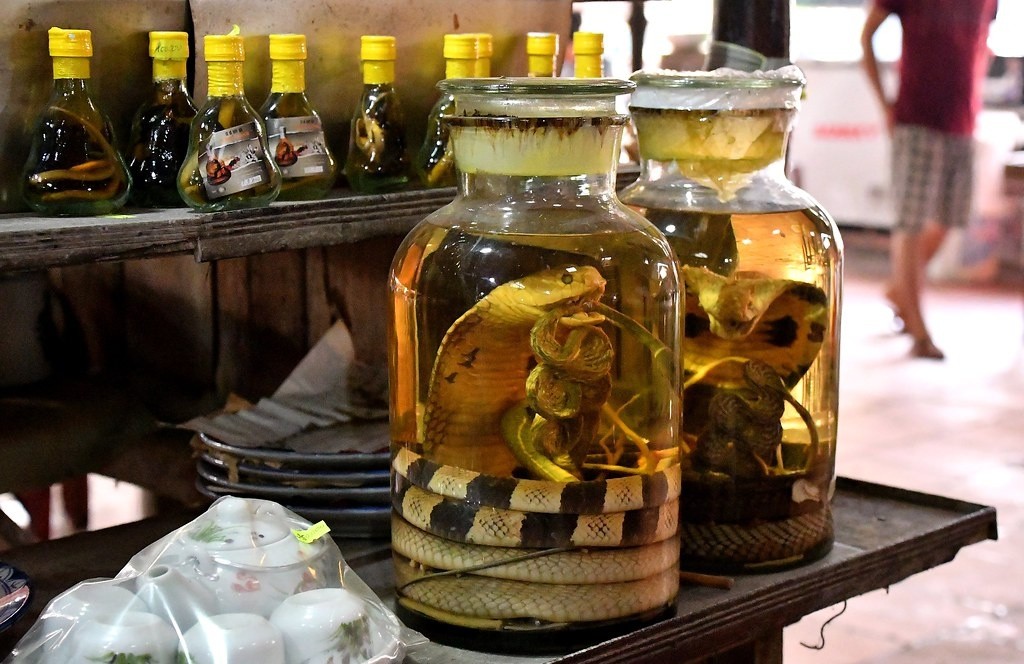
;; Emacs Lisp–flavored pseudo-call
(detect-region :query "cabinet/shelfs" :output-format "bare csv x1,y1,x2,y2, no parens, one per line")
0,166,1000,664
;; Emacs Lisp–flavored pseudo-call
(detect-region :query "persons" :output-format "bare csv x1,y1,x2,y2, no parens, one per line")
861,0,998,359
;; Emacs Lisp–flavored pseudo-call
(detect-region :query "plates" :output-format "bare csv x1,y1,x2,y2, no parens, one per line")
192,405,400,532
1,560,32,634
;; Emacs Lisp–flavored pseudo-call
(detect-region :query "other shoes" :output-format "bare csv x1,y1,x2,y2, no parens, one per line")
885,292,906,333
913,338,944,360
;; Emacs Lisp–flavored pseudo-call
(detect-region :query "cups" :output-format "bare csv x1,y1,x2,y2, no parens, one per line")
40,584,386,664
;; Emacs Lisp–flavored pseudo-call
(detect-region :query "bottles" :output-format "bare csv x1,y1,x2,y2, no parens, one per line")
20,26,133,218
414,36,477,190
573,31,606,79
527,33,560,78
256,33,336,200
176,33,284,209
384,77,685,648
613,72,847,579
463,31,493,78
338,35,413,194
129,31,203,206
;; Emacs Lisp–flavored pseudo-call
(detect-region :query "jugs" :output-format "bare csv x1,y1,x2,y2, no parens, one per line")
143,507,333,628
